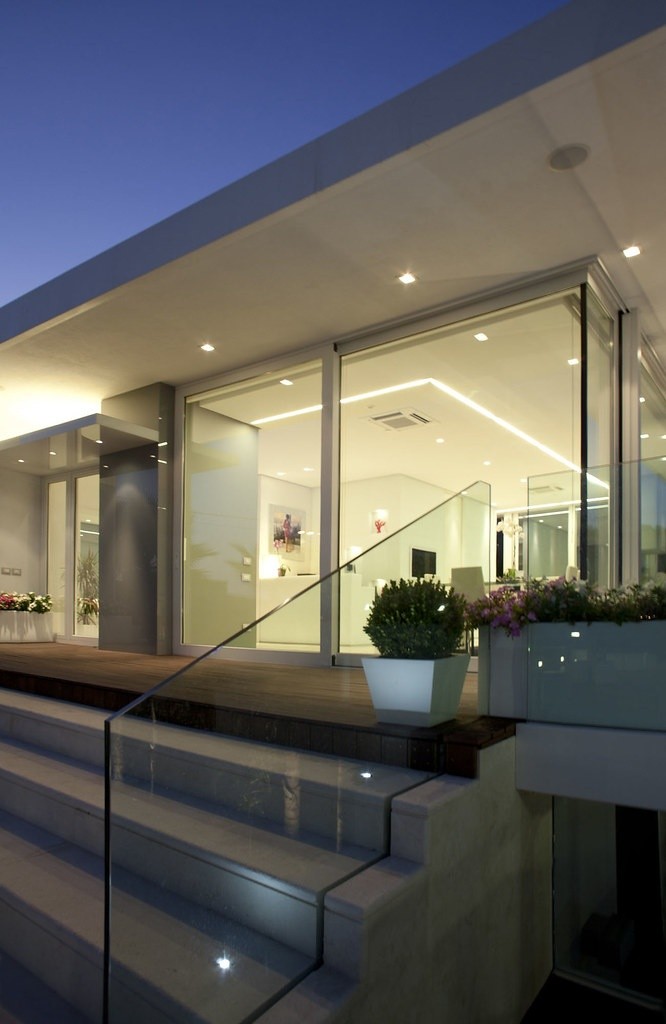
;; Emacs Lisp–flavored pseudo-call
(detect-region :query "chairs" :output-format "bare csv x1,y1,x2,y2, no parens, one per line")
452,566,487,656
565,566,578,582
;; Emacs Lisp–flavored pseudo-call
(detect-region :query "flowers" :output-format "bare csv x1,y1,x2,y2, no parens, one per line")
0,590,53,614
456,574,666,641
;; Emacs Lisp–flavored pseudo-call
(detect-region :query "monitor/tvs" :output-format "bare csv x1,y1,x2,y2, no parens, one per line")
412,548,436,574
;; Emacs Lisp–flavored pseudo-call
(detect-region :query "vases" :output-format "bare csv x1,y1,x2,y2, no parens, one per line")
0,611,54,642
476,619,666,733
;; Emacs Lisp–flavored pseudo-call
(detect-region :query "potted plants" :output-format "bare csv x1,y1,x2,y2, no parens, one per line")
360,572,472,728
60,545,99,638
277,561,291,577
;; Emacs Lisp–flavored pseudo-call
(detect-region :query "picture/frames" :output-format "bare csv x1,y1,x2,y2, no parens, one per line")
268,503,306,562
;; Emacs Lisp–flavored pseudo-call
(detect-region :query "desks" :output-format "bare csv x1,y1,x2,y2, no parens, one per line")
437,581,525,592
